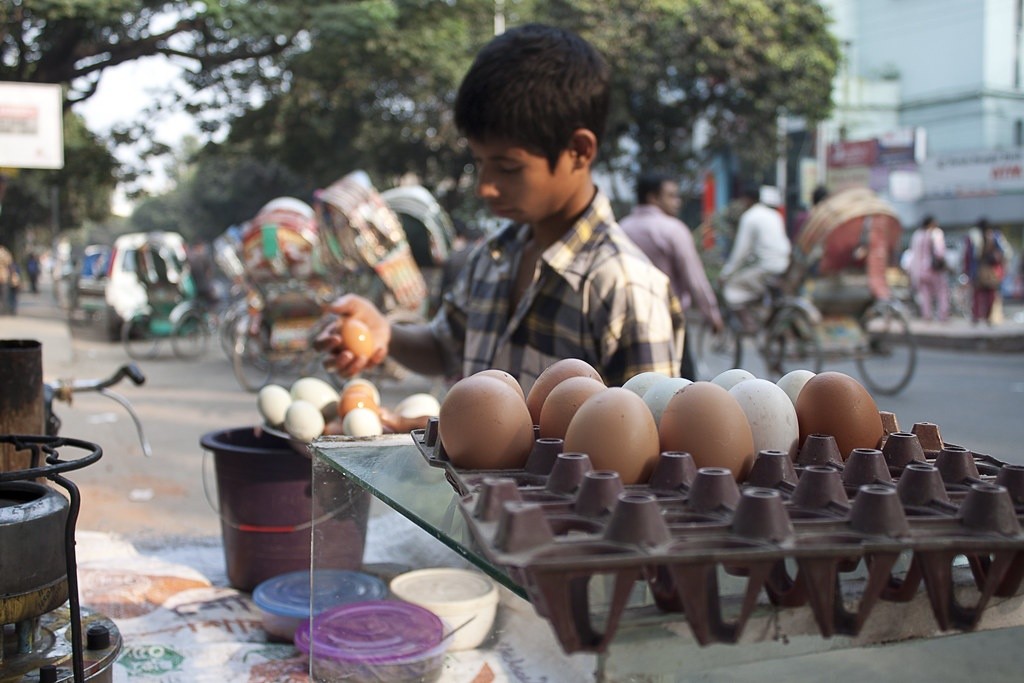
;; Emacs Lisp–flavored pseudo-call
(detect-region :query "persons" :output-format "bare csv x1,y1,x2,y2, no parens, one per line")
28,254,40,291
615,168,725,381
963,217,1006,324
717,186,830,333
313,23,686,401
909,215,954,321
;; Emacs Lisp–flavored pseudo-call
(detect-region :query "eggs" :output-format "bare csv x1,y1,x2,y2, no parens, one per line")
332,320,373,357
439,357,885,485
258,376,442,441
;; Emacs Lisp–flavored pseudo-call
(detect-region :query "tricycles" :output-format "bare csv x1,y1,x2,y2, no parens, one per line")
122,170,457,396
696,188,918,396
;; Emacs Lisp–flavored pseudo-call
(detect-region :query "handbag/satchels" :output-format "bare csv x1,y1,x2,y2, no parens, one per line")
928,240,948,270
987,242,1004,265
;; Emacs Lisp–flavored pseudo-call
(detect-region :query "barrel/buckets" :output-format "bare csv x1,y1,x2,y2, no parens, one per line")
200,426,372,590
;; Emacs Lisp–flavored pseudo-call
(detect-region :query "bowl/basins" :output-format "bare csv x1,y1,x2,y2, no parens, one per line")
388,568,501,652
294,599,454,683
252,566,385,640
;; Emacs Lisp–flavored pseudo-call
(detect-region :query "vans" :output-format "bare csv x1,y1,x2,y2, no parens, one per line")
107,231,188,340
